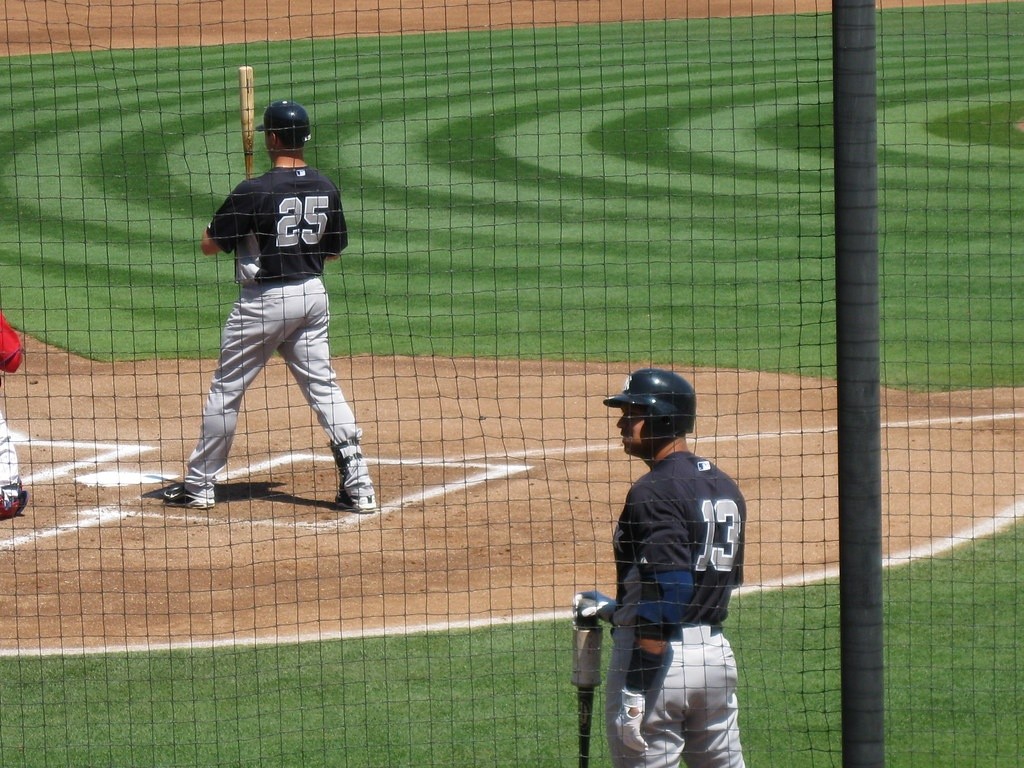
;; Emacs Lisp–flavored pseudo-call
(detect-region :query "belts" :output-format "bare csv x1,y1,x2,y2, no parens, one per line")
669,624,724,641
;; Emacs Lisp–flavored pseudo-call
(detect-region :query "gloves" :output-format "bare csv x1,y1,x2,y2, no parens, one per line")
614,687,651,752
571,590,616,624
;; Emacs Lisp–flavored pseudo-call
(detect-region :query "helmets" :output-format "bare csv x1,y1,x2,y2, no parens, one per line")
603,368,697,444
255,101,311,143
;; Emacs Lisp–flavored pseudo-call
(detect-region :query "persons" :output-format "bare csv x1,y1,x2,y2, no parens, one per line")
574,367,748,768
161,100,377,512
0,305,28,520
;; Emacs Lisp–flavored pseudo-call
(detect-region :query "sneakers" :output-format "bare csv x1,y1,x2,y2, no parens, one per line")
163,485,215,508
335,493,376,513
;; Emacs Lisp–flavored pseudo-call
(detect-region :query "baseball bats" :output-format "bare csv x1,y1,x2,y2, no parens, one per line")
567,598,602,768
236,64,255,180
